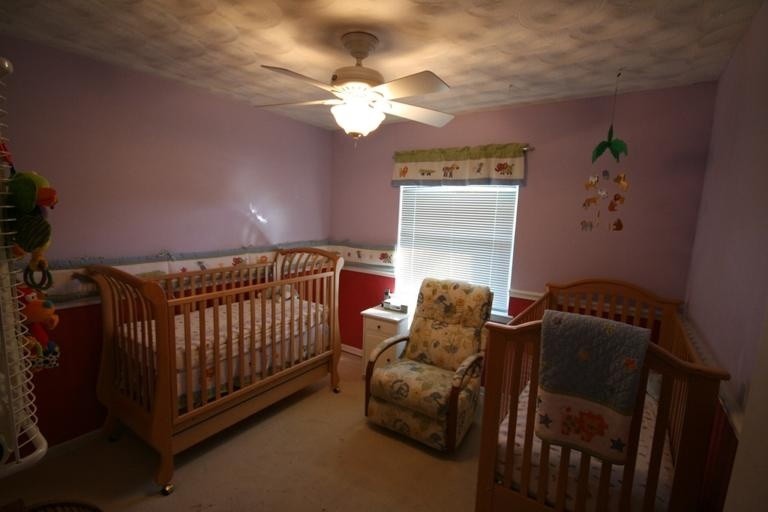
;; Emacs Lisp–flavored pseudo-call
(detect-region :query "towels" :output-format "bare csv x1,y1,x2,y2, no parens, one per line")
535,309,652,465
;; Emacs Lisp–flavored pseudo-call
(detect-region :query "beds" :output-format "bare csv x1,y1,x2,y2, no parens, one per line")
475,280,730,512
71,248,344,495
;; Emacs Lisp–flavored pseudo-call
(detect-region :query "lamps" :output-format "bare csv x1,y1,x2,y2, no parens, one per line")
331,93,385,141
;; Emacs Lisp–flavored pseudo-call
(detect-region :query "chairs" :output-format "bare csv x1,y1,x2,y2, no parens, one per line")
365,278,494,453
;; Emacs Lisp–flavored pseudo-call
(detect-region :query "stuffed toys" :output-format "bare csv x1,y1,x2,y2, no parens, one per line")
14,285,59,369
7,160,57,292
256,283,298,302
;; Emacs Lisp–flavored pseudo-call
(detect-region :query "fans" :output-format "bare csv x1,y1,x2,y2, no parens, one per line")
254,32,455,130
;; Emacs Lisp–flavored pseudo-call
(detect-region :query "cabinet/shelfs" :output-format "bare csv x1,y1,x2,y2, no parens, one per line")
361,305,409,379
